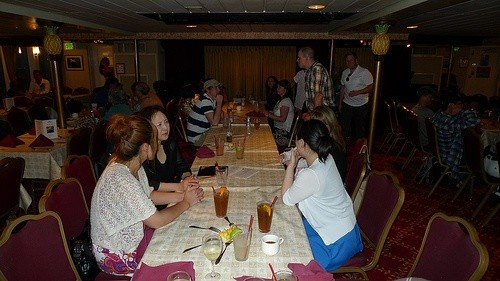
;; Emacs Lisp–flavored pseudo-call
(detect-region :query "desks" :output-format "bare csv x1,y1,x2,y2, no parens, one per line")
478,117,500,149
131,101,316,281
0,128,72,180
62,93,89,99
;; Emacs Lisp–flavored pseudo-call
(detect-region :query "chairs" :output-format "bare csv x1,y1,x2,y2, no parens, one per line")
163,98,197,167
407,213,490,281
331,170,407,281
343,137,370,199
276,111,300,153
379,98,500,228
0,87,131,281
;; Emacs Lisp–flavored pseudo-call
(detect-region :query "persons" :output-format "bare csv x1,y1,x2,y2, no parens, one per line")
288,47,335,144
412,86,500,188
264,76,281,111
91,114,205,274
7,97,33,136
29,99,48,120
310,104,345,150
166,78,225,169
27,70,51,97
89,62,164,121
339,52,373,140
282,118,363,273
137,106,200,211
263,80,295,146
6,77,26,97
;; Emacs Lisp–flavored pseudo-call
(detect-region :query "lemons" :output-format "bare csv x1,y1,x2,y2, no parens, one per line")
220,187,225,196
263,205,271,216
236,147,243,152
230,228,242,240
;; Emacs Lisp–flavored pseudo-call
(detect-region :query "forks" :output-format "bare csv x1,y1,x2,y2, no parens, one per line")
184,239,218,253
189,226,221,233
224,217,234,226
215,240,233,264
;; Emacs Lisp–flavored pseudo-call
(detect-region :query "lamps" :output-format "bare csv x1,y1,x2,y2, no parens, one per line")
371,23,391,61
43,26,62,60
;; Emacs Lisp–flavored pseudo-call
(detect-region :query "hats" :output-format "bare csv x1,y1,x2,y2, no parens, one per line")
203,79,224,92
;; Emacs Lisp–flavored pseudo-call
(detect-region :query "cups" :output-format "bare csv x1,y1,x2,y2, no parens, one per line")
166,271,192,281
272,270,298,281
233,224,252,262
261,234,284,255
234,142,245,159
71,113,78,118
227,106,232,118
213,188,229,218
256,200,275,233
94,118,98,124
213,135,225,156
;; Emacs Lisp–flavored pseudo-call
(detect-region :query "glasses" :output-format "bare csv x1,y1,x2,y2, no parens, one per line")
346,72,353,82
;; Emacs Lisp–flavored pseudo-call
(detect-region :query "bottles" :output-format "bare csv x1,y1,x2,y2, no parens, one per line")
241,98,244,107
246,113,250,127
254,101,258,111
226,121,232,142
236,103,241,114
233,98,236,110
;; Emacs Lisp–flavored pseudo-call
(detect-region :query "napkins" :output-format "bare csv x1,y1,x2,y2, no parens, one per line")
194,143,216,158
132,261,196,281
28,134,55,147
0,134,25,148
246,110,266,119
287,260,336,281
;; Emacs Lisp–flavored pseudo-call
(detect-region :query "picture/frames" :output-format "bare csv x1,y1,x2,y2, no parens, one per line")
116,62,126,75
64,55,84,72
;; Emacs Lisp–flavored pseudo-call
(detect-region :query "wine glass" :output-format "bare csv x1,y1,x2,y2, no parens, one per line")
202,233,223,281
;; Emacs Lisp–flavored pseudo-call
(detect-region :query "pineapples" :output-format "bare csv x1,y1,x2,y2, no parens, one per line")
43,25,62,54
371,25,389,55
